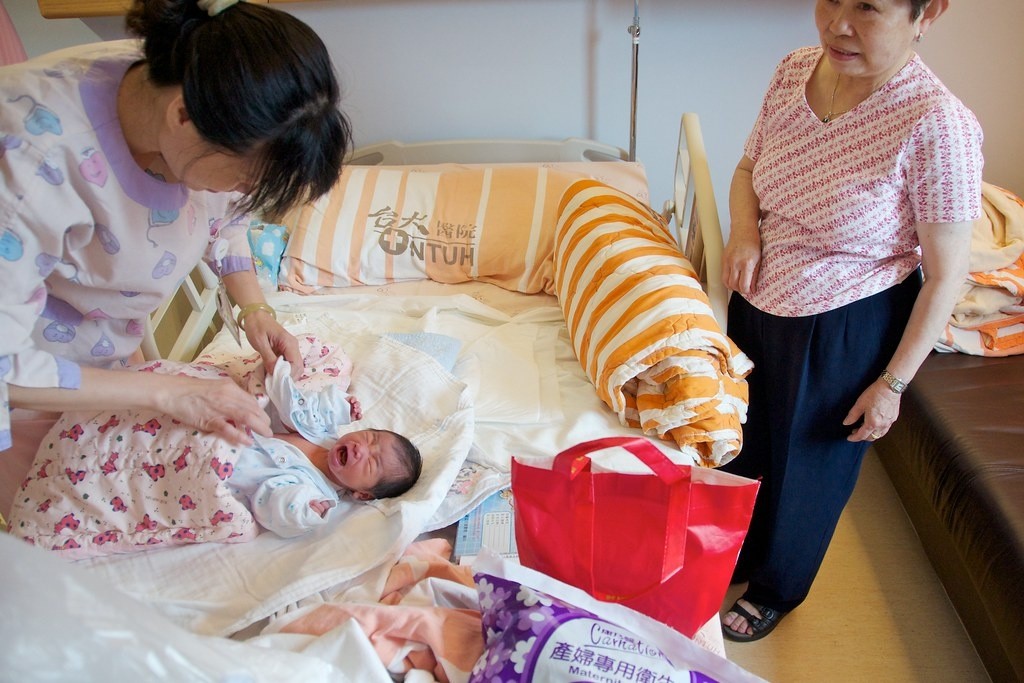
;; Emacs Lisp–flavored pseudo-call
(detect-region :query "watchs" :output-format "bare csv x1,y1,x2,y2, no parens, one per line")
882,370,907,393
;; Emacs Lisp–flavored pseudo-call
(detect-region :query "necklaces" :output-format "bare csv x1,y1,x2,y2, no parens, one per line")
822,73,851,122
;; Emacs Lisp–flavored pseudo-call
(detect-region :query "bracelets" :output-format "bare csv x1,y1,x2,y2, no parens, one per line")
237,303,276,331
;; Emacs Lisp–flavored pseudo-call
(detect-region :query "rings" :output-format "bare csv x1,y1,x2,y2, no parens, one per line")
871,434,879,439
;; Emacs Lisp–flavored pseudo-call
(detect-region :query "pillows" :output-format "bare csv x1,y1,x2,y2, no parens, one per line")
273,165,588,295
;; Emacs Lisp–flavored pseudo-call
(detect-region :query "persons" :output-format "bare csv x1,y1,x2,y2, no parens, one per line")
10,356,424,538
722,0,985,641
1,0,359,448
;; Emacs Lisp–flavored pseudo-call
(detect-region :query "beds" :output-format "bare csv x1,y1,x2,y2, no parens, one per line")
1,112,725,683
873,350,1024,682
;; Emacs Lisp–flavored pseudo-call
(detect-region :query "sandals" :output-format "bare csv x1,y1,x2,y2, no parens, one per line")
721,596,789,642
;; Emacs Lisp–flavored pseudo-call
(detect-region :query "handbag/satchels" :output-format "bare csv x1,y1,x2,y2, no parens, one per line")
510,437,762,641
